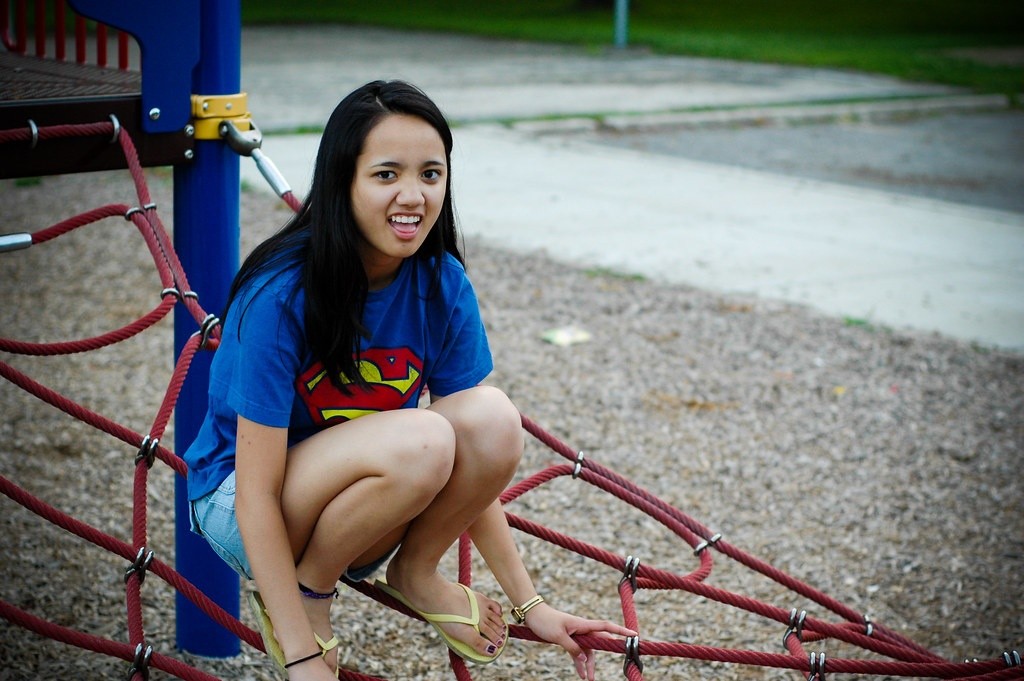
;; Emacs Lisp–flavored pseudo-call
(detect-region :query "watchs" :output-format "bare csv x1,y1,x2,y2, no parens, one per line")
511,594,544,623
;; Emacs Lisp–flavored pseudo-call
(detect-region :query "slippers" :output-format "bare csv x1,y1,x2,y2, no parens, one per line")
246,583,340,681
373,576,508,665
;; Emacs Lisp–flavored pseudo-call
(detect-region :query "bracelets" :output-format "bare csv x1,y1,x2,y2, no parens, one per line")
284,651,323,668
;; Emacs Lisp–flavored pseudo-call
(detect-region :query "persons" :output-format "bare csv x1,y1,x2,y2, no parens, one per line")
183,78,638,681
